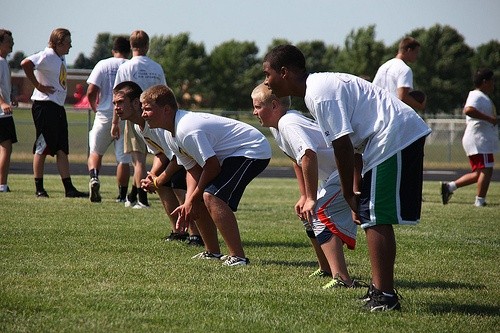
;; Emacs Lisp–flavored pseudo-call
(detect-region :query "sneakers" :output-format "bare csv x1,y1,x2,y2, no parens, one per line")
115,197,126,202
191,250,228,262
359,284,402,311
131,200,147,209
222,255,249,267
308,268,332,280
36,189,56,197
125,195,136,208
162,230,189,242
0,184,12,192
441,181,454,205
89,177,101,203
186,235,204,247
322,273,363,290
356,280,379,302
66,188,89,196
475,201,487,207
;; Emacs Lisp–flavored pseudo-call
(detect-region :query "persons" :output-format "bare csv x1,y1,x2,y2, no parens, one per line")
111,81,206,247
0,30,18,192
371,37,427,110
110,30,167,208
21,28,90,197
251,83,361,289
264,44,432,310
140,86,271,266
86,36,134,203
441,69,500,207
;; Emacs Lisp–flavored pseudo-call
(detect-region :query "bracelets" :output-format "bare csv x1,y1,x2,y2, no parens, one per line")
154,179,158,188
355,192,361,195
